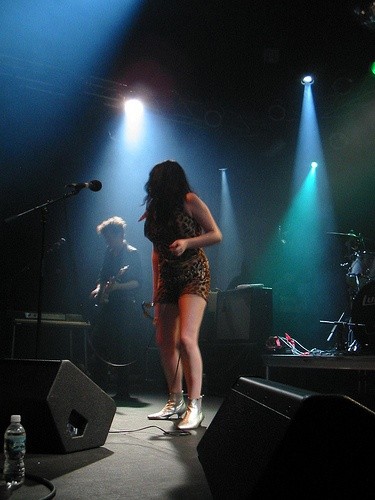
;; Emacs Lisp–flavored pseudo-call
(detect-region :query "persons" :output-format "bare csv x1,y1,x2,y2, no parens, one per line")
137,161,223,430
89,216,143,401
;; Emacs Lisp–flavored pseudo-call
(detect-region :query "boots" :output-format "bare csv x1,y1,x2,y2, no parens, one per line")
178,395,204,429
147,391,187,419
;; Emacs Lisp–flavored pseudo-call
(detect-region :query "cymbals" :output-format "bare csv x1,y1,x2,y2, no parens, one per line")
322,229,369,244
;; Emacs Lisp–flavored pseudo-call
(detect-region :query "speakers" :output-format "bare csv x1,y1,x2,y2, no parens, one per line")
202,286,375,500
0,318,117,454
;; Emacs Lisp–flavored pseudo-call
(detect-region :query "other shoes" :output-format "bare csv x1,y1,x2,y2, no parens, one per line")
111,392,130,400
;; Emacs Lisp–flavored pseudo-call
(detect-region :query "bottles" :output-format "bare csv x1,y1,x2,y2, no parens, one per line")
2,414,26,490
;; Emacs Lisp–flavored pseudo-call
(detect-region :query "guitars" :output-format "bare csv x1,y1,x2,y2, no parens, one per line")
92,264,133,311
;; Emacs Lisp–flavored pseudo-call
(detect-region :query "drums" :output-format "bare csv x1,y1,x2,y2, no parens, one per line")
350,279,375,355
344,251,375,292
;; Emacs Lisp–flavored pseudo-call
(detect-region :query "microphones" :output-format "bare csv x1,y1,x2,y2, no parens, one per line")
68,179,102,192
